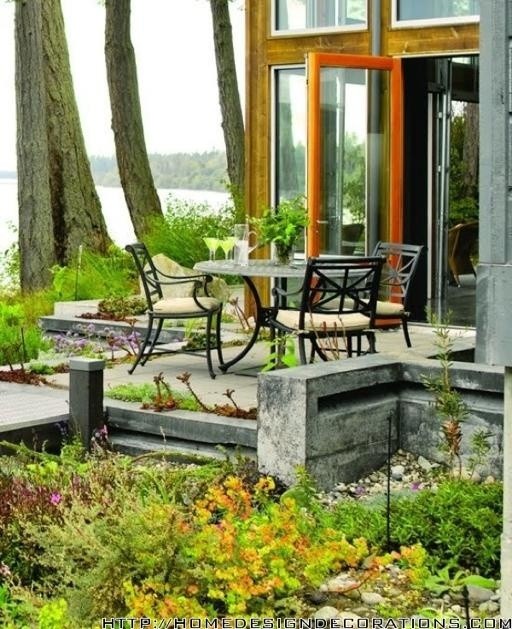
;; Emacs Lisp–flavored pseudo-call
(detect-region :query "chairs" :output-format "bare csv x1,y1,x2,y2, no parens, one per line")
444,221,480,289
119,240,423,380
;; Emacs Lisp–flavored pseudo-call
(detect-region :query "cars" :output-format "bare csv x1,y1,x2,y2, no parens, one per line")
234,223,259,265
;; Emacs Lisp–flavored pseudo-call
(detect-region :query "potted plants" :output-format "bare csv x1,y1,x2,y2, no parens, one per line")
248,198,311,267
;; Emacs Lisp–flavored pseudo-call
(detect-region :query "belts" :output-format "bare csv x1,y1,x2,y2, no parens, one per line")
202,235,239,268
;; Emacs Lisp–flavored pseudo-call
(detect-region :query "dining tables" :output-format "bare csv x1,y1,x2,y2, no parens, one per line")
248,198,311,267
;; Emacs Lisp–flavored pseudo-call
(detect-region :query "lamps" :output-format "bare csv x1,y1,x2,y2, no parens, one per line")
444,221,480,289
